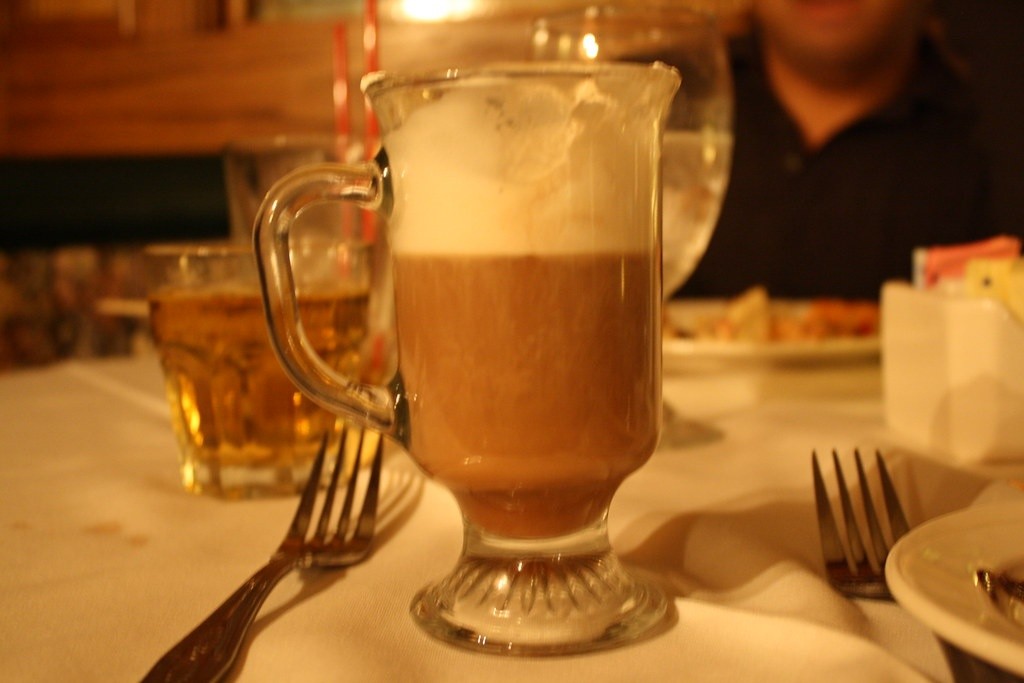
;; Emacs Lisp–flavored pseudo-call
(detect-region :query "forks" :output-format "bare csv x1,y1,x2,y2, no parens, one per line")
811,448,999,683
139,421,385,683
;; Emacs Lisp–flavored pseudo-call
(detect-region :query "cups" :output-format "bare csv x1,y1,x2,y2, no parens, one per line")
146,239,371,501
373,1,734,306
250,61,682,657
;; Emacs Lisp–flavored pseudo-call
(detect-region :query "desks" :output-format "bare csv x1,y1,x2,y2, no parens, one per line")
0,349,956,683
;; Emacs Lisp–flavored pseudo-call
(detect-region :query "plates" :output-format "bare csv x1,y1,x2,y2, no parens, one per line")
885,498,1024,679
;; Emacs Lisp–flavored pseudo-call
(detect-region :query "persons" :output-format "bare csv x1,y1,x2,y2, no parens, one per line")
609,1,1024,302
